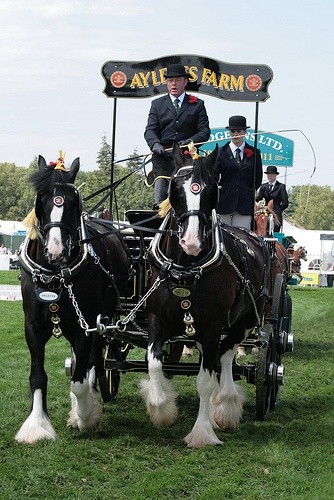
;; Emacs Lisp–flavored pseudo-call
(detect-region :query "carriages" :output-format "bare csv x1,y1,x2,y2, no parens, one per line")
9,54,301,449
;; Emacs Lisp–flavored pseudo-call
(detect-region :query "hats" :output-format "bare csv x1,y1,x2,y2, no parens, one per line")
164,64,194,79
264,166,279,175
225,116,250,130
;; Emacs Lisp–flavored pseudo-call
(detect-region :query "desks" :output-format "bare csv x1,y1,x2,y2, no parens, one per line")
0,254,15,271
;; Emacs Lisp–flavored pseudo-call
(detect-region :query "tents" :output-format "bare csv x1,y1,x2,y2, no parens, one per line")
0,220,27,251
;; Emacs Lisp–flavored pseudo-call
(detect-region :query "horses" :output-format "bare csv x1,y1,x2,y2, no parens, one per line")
11,156,137,446
140,140,267,448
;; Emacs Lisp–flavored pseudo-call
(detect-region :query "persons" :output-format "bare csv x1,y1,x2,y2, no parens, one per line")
144,64,211,210
255,166,289,233
0,243,19,269
213,116,262,231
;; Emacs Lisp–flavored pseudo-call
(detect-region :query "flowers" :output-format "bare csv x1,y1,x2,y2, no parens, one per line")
186,93,199,105
244,148,255,160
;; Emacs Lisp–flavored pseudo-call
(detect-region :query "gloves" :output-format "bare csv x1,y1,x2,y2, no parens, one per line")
178,140,191,145
152,143,165,157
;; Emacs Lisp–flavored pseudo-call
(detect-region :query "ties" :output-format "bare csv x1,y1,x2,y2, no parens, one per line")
235,149,242,166
268,185,273,194
174,99,180,117
